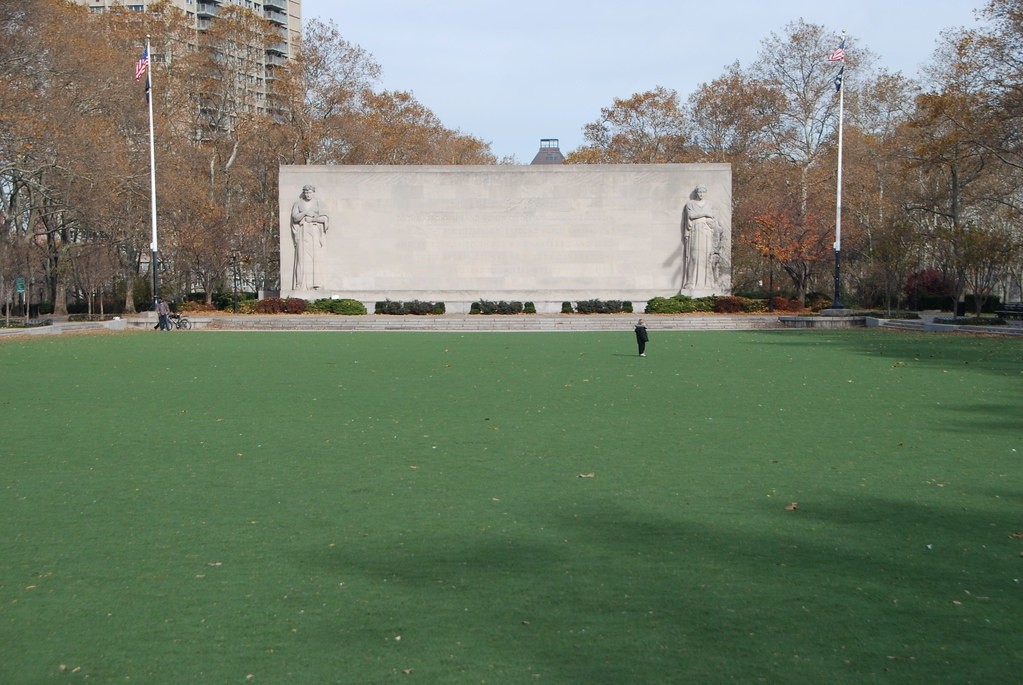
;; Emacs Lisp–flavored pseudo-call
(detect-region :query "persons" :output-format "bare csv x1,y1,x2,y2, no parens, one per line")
292,186,330,290
683,185,724,289
635,319,649,356
153,297,171,331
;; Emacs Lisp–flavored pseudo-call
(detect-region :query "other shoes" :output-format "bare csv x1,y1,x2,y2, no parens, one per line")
640,353,646,357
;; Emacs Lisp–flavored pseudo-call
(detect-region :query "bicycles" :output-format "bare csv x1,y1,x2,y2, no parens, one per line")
163,312,192,331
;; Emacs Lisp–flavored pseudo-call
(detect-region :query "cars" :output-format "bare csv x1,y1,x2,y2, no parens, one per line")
993,302,1023,320
